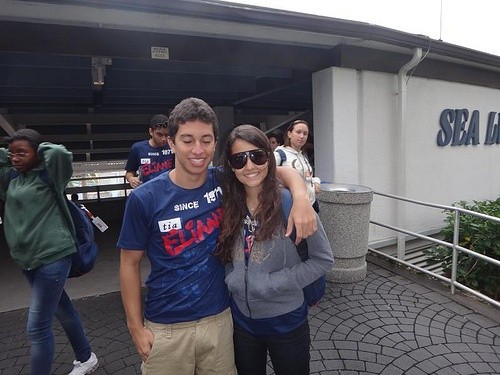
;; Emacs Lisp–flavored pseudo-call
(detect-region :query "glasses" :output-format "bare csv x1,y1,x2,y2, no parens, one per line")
289,120,309,130
155,122,168,129
7,152,35,159
228,149,270,170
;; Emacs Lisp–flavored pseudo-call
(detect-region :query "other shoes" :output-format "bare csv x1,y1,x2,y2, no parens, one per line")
68,352,100,375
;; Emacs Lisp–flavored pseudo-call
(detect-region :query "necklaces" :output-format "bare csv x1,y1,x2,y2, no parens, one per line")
250,208,255,212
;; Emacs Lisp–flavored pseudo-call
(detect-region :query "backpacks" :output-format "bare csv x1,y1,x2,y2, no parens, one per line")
0,167,109,279
279,187,327,307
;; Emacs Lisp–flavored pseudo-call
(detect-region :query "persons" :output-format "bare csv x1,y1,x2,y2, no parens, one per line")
269,131,282,151
116,97,319,375
125,115,175,188
273,121,320,214
212,124,335,375
0,129,99,375
